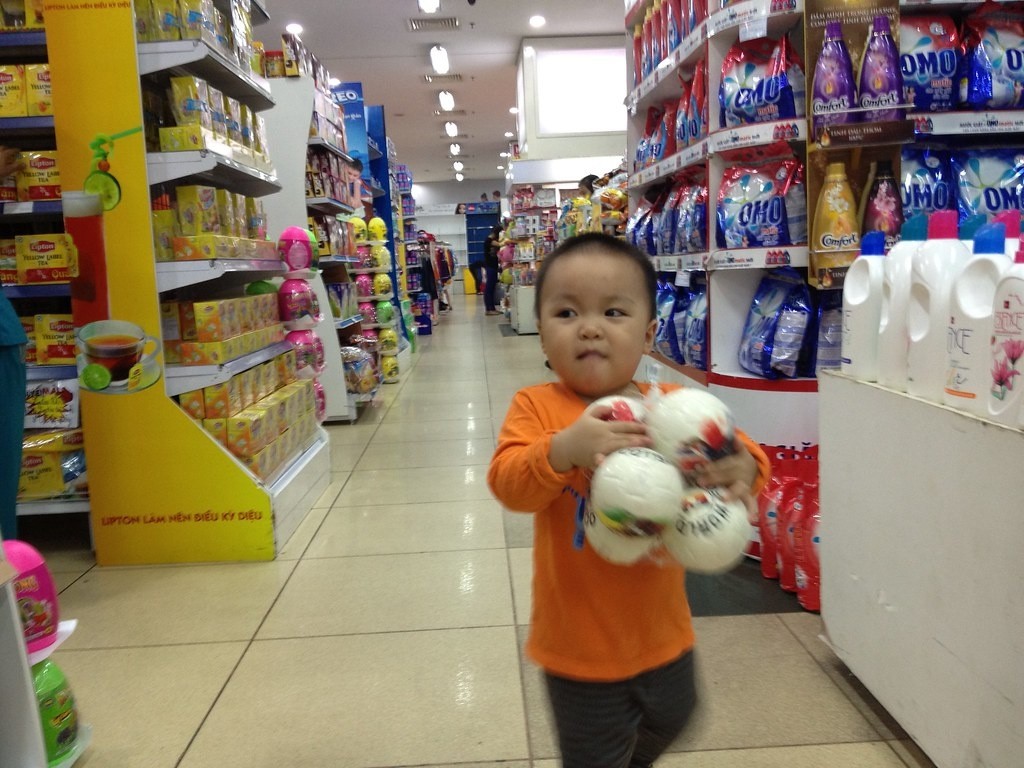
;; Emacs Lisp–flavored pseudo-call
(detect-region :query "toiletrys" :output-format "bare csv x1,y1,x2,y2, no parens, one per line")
840,209,1024,431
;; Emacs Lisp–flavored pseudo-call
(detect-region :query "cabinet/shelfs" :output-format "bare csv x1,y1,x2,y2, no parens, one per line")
0,0,455,768
464,0,1024,768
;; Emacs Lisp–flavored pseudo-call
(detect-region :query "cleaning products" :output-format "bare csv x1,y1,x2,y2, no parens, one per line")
626,0,1024,381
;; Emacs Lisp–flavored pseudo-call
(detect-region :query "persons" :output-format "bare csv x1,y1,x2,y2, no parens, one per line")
483,225,505,316
345,157,366,210
577,174,599,199
486,231,772,768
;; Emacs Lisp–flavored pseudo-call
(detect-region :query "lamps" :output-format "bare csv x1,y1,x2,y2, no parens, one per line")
428,44,451,75
438,90,455,113
453,162,464,173
444,120,459,138
448,143,461,156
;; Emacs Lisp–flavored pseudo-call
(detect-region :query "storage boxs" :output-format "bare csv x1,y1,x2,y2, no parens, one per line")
292,377,315,411
283,350,299,386
239,368,254,410
289,422,300,452
203,418,227,447
308,408,318,437
179,388,206,418
300,413,309,443
265,439,280,474
247,403,279,444
273,356,284,389
226,414,265,457
263,358,275,398
274,387,299,428
261,395,287,435
283,384,308,417
252,364,265,404
278,429,290,461
240,448,267,483
205,375,241,419
